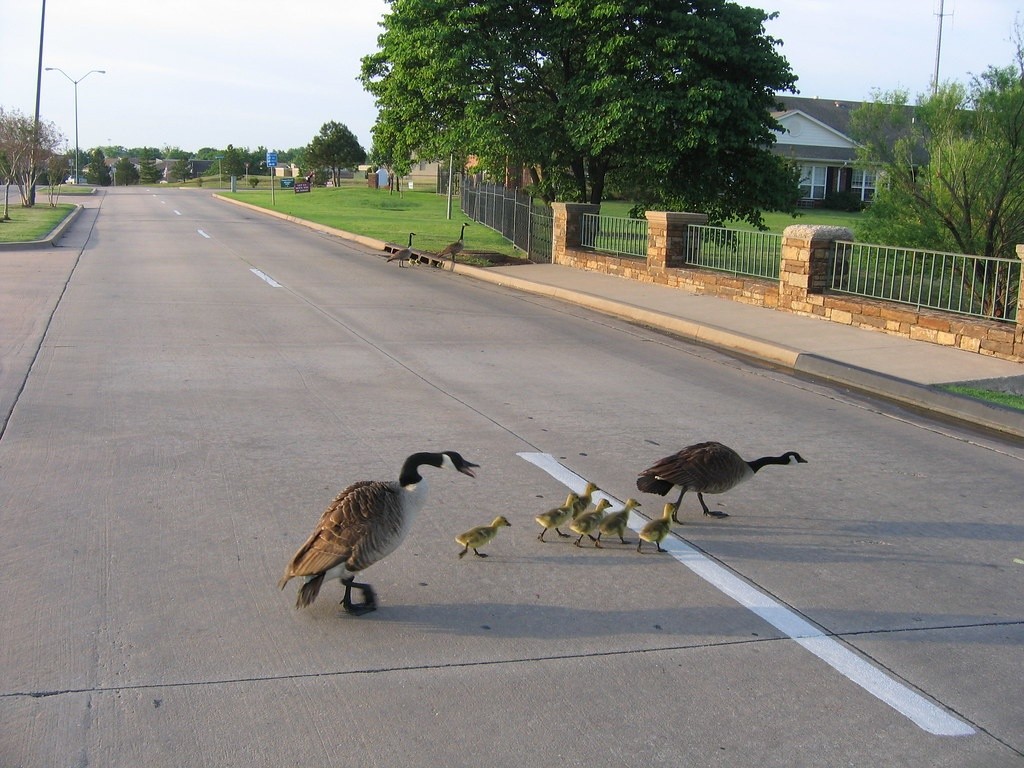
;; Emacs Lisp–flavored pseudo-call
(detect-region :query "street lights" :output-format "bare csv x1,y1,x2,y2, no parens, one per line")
45,68,106,185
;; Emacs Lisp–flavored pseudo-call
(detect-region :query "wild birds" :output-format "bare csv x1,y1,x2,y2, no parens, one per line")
277,437,812,618
436,221,471,262
386,222,422,269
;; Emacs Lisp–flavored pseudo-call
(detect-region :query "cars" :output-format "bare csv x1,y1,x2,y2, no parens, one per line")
365,167,378,179
